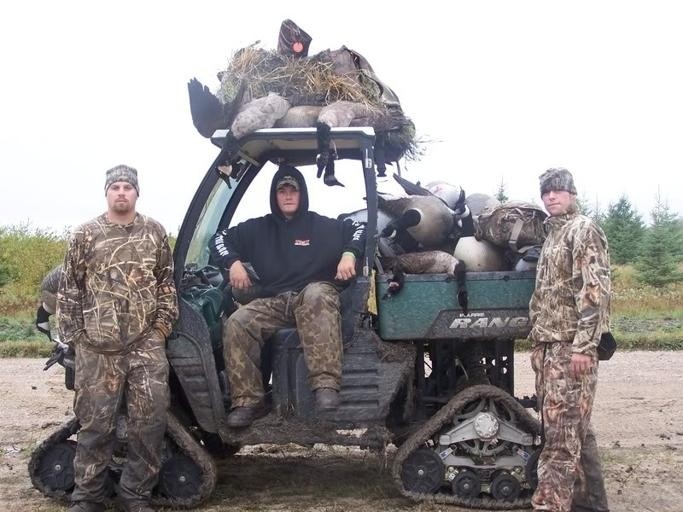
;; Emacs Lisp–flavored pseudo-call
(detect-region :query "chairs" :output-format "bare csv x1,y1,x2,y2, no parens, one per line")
272,274,371,351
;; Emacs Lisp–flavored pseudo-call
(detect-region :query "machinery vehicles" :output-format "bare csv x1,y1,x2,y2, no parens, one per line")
23,124,548,507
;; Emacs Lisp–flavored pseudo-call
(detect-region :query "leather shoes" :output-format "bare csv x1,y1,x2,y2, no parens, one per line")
65,500,108,512
225,397,271,428
313,387,341,411
115,498,157,512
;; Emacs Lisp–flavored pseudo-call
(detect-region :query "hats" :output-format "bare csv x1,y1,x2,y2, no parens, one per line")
103,164,140,197
538,167,578,200
275,174,301,193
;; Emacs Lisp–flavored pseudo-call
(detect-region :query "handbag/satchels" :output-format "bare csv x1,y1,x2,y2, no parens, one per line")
596,331,618,362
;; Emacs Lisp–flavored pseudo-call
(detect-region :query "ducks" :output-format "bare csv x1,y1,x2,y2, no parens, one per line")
336,174,541,317
35,263,67,343
186,75,390,191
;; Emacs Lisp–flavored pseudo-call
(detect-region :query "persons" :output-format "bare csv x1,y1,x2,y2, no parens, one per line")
56,166,179,511
208,165,356,427
528,170,610,512
